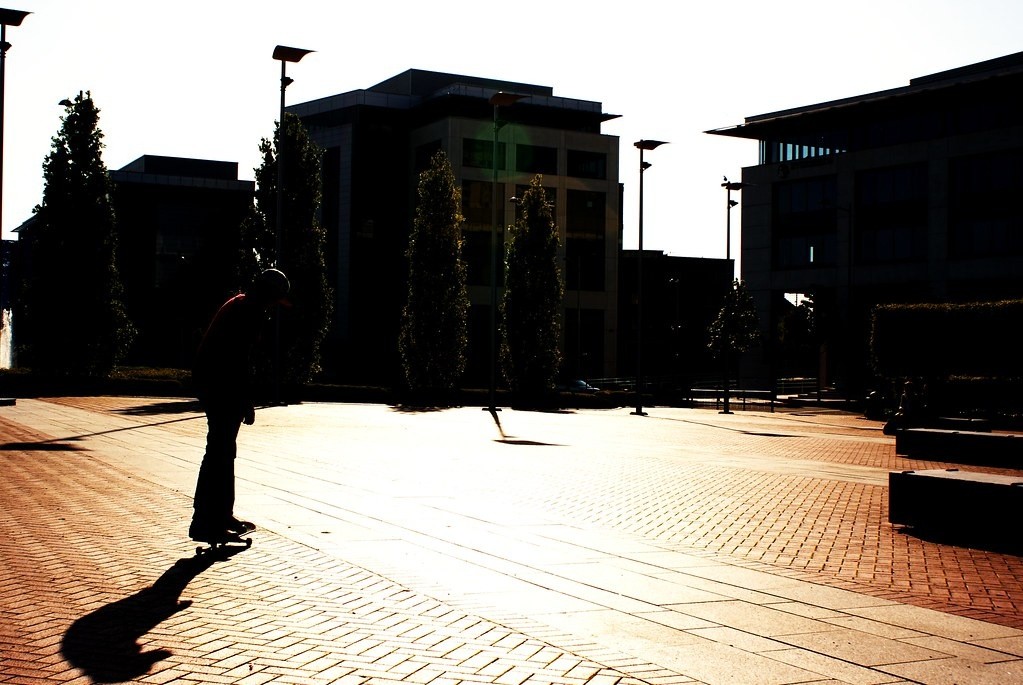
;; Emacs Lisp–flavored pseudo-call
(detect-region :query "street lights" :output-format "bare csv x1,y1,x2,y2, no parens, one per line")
718,180,755,414
630,139,670,415
273,45,320,134
490,91,534,265
0,9,34,328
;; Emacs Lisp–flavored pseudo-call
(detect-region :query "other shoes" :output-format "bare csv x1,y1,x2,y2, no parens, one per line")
189,518,241,543
227,512,256,533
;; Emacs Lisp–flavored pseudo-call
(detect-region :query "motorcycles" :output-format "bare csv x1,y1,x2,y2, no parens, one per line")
883,377,939,434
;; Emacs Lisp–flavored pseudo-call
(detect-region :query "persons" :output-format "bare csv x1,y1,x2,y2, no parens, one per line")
189,268,289,540
883,378,914,435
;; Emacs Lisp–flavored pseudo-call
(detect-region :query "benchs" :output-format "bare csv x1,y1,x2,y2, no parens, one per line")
583,376,821,407
889,415,1023,554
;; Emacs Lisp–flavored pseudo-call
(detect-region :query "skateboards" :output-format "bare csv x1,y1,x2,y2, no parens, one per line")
192,521,257,559
